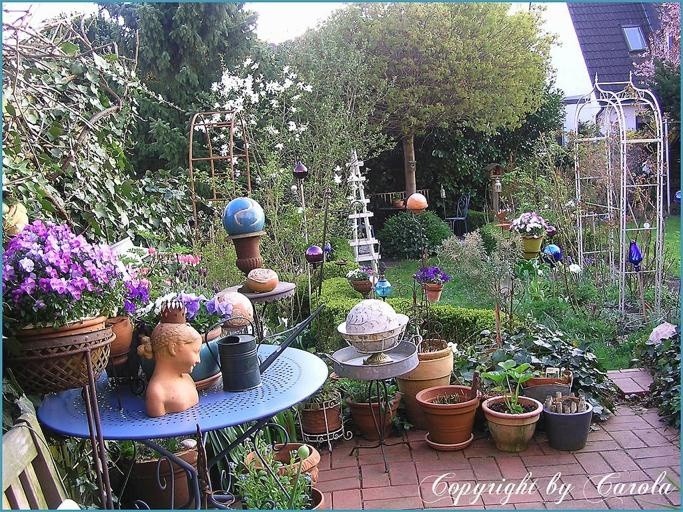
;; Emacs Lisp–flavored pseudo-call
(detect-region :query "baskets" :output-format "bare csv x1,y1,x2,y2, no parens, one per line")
5,323,112,396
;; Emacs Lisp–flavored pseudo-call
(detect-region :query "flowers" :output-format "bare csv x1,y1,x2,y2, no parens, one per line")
133,293,233,331
124,265,151,312
509,212,556,237
346,266,372,280
413,267,448,284
2,221,128,331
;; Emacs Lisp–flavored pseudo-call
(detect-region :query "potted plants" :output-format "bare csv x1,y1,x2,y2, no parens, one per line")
122,437,198,510
416,359,592,451
398,338,453,430
233,443,324,510
297,378,402,439
392,193,404,207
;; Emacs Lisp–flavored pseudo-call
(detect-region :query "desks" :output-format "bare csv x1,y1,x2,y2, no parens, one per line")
333,340,419,454
220,283,295,342
35,344,329,510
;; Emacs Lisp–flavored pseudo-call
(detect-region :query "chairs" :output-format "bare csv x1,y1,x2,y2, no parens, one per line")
1,413,65,510
445,193,471,236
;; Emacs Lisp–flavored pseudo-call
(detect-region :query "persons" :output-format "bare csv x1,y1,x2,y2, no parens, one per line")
144,298,206,416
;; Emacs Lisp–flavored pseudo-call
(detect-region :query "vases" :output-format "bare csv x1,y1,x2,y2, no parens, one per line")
17,315,107,391
105,316,132,366
354,281,372,298
524,236,542,260
427,285,443,301
139,327,222,390
